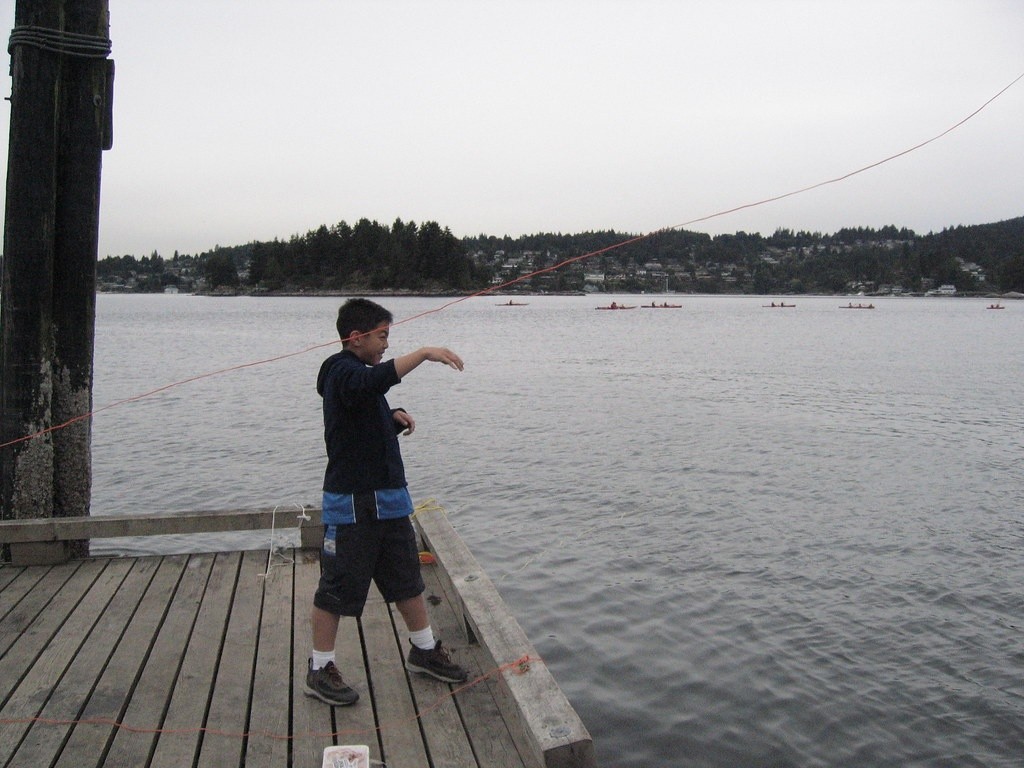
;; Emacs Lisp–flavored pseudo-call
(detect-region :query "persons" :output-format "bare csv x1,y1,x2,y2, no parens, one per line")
302,297,470,707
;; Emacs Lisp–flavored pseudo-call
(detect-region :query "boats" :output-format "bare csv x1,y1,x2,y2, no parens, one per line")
762,305,797,307
642,305,684,308
495,302,527,306
986,306,1004,310
839,305,874,309
596,306,636,309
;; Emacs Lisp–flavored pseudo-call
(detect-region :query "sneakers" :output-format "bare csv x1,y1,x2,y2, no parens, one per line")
304,656,359,706
405,637,467,682
